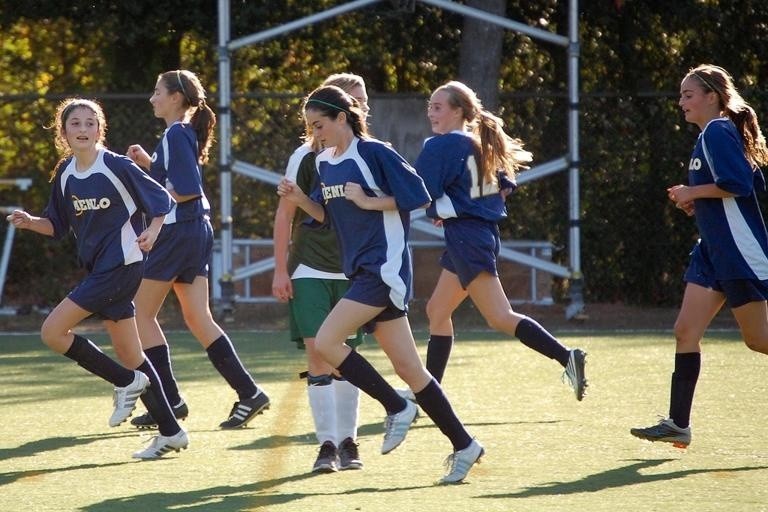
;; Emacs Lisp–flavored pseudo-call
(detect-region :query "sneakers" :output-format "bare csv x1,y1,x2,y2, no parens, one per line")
439,438,485,483
562,347,588,401
107,369,152,428
312,440,339,473
133,430,189,460
218,387,270,428
339,437,363,470
130,397,191,428
382,388,421,455
629,418,694,450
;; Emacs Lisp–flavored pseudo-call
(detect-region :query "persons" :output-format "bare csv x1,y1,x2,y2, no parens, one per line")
125,70,270,432
629,64,766,450
271,72,379,475
393,81,589,416
276,84,484,486
5,96,190,461
